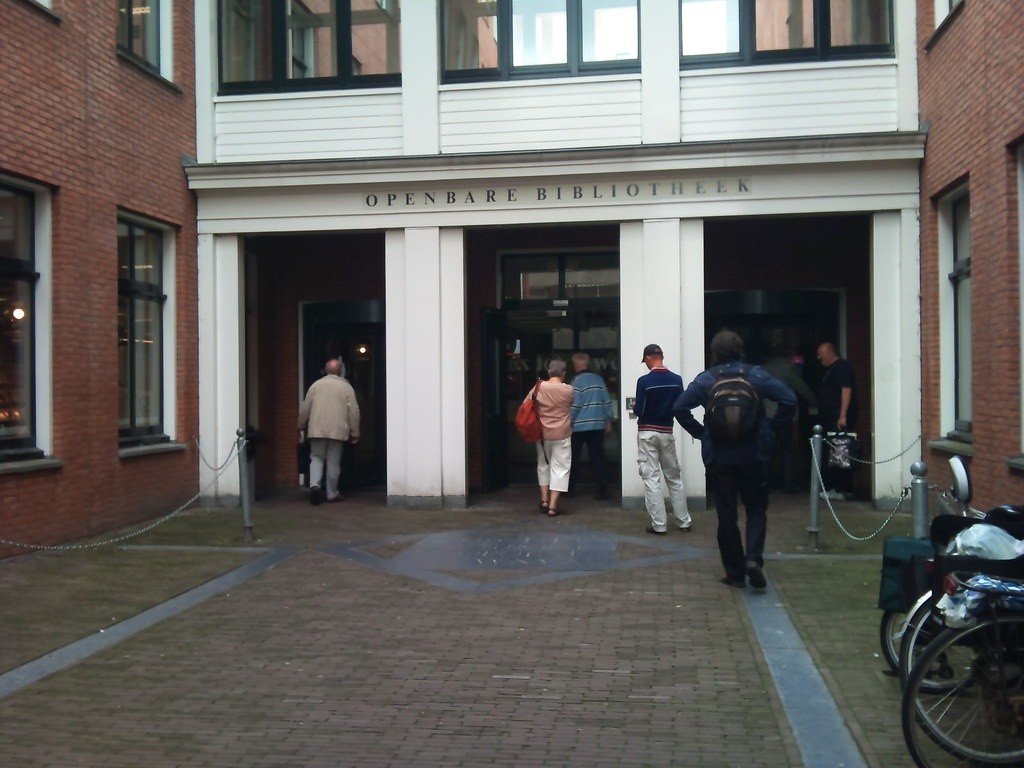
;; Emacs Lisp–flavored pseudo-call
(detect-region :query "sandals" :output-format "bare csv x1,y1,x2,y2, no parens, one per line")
540,501,559,518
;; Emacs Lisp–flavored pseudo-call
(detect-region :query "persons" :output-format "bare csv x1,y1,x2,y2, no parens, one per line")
568,353,613,498
817,343,856,501
673,330,797,589
633,344,692,534
523,360,574,516
298,359,360,505
757,354,816,477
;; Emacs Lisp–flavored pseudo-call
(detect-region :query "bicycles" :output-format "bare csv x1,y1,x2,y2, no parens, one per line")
877,505,1024,768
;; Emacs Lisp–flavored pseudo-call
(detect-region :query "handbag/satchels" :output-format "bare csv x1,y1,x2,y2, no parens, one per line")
828,427,853,470
516,400,544,444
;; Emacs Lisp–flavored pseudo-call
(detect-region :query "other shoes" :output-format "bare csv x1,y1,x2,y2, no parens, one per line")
721,571,745,588
820,488,845,501
679,525,691,532
745,561,767,589
310,486,323,505
645,526,668,535
596,489,613,501
328,494,343,503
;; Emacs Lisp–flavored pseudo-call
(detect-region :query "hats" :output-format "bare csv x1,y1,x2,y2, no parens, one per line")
641,344,663,363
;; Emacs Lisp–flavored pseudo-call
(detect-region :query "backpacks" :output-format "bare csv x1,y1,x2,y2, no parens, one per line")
708,364,761,450
